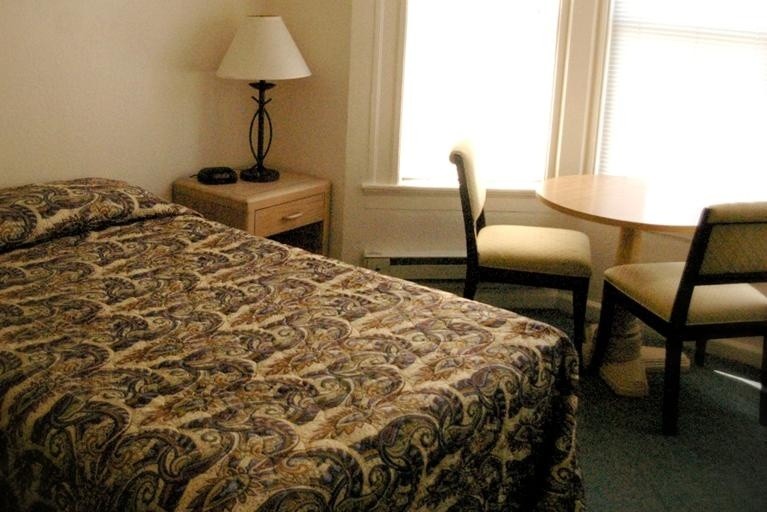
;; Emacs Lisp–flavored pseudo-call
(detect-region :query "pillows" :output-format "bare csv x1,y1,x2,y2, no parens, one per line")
0,177,203,250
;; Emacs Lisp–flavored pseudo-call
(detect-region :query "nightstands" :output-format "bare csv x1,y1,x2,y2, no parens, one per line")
172,170,332,256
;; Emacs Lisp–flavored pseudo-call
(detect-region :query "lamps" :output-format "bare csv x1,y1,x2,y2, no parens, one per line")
216,15,312,183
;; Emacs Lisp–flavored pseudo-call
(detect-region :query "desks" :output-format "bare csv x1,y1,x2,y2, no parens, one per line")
536,175,699,395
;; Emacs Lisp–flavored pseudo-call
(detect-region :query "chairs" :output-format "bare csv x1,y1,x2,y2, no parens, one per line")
449,139,593,368
587,201,767,439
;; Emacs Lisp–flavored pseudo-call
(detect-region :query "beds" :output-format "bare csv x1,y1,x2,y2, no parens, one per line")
0,215,589,512
